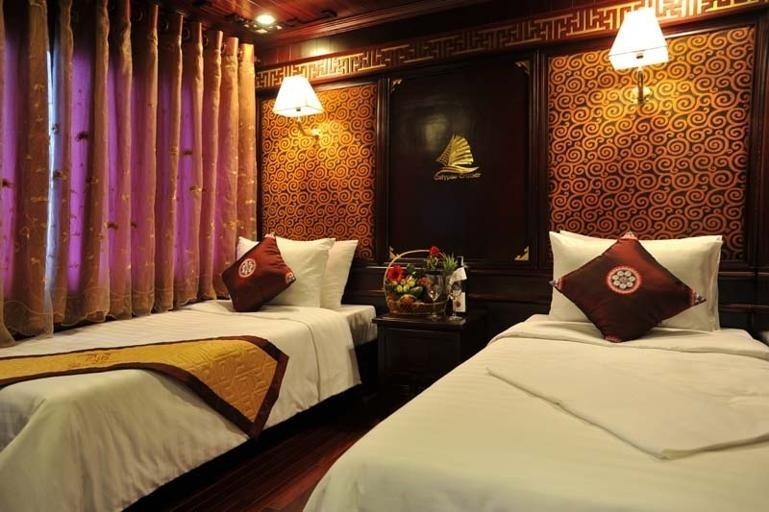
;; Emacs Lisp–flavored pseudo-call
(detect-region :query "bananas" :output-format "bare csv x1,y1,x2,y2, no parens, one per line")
392,275,415,293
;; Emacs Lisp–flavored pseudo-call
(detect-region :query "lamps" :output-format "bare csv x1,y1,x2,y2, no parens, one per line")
609,8,668,102
272,75,323,142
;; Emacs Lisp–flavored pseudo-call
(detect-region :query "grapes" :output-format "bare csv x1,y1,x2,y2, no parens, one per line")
416,276,429,288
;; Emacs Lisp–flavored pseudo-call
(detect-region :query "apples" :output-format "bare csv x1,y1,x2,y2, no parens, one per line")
387,263,402,282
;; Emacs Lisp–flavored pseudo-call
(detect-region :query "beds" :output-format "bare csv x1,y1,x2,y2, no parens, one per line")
301,314,768,512
0,299,377,511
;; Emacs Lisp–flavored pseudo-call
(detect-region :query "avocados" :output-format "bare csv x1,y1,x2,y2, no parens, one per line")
400,293,417,304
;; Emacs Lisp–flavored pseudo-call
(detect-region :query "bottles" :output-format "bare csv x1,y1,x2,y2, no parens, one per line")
451,256,467,317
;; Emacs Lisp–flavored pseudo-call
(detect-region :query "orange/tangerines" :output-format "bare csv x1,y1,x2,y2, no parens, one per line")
409,286,424,296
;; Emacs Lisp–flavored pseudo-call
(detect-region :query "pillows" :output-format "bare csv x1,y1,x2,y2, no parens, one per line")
276,236,359,310
237,237,336,308
549,229,706,343
222,232,296,311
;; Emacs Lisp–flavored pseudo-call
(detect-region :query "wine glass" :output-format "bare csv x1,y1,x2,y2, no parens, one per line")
426,275,443,320
446,275,462,321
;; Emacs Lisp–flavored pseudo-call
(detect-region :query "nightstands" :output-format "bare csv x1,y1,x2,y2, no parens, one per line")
372,308,489,415
